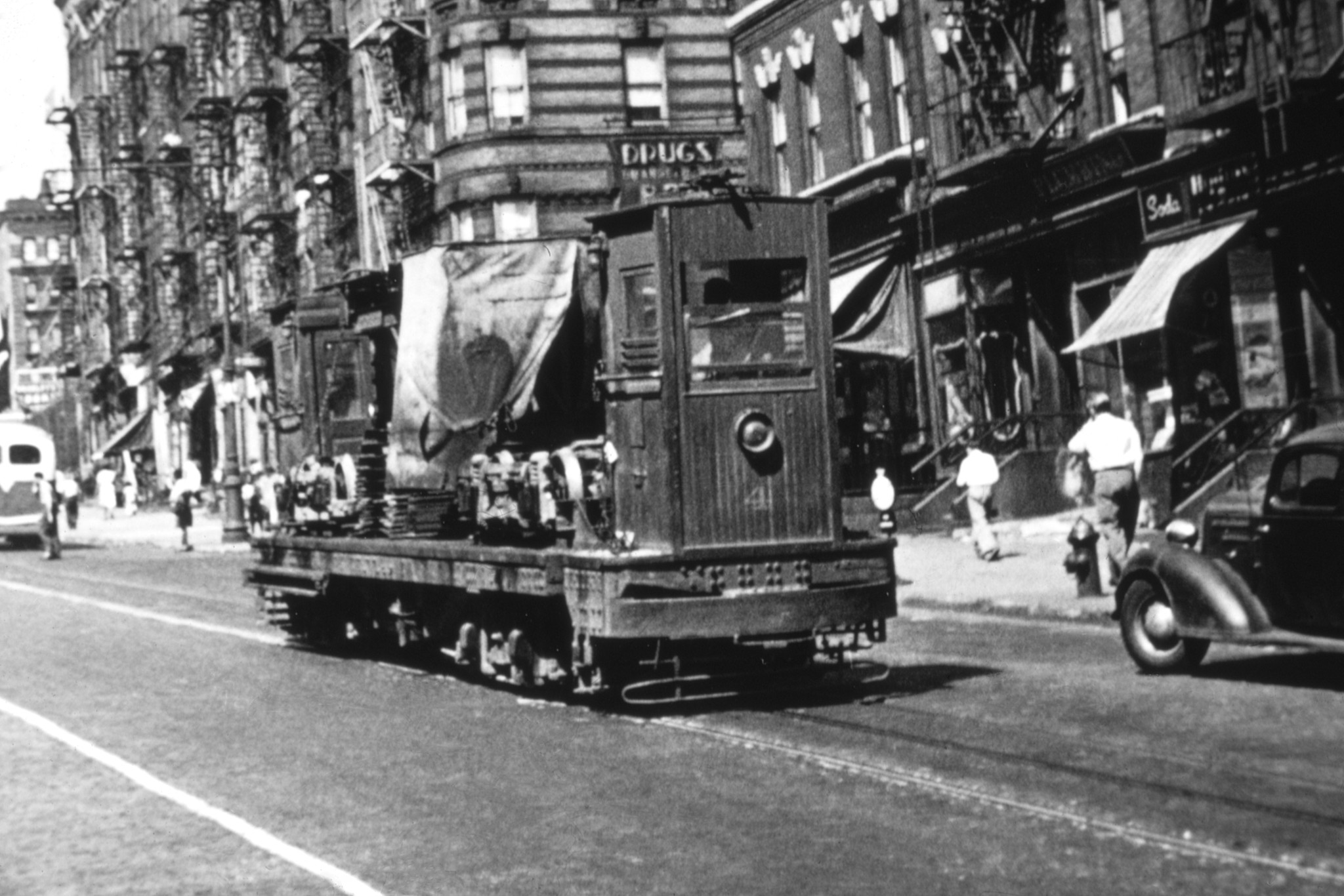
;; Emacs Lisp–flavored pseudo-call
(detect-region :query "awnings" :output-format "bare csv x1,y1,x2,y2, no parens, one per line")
1059,212,1249,352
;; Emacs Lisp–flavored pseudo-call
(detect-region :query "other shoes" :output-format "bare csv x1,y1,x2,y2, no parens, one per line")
42,553,59,559
185,544,192,551
984,548,998,560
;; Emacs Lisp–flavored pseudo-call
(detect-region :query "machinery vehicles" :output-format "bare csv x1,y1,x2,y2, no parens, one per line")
244,195,897,710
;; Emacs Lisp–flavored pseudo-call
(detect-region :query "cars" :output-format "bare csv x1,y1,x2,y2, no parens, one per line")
1118,421,1344,675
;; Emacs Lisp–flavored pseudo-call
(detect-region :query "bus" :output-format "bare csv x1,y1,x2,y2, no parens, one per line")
0,421,57,547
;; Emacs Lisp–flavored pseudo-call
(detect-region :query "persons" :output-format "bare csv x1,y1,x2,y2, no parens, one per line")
1055,441,1090,517
1066,391,1143,588
1177,369,1234,488
56,446,285,551
956,438,999,563
32,472,59,562
